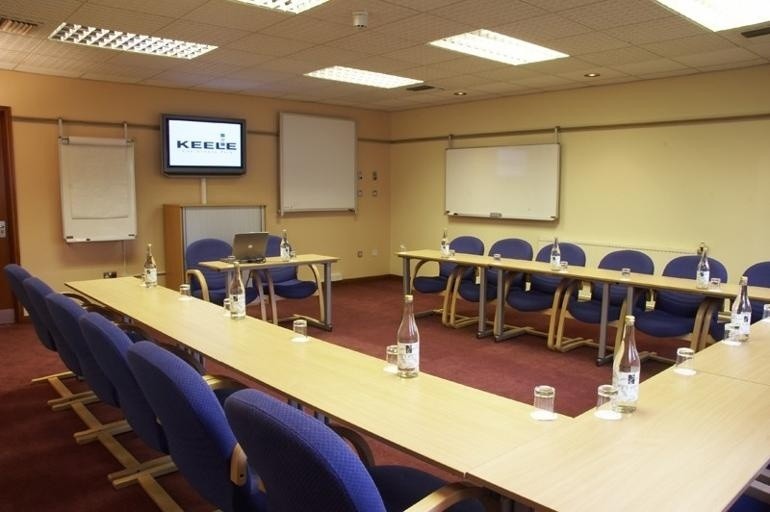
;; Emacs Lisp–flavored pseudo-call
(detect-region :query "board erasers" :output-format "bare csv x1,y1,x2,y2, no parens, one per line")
490,212,502,218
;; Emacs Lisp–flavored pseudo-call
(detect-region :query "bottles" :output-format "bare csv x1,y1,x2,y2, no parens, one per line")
611,315,640,414
441,228,450,258
280,229,290,263
697,248,709,289
551,238,560,271
143,244,157,288
732,277,753,343
229,262,246,321
397,295,420,379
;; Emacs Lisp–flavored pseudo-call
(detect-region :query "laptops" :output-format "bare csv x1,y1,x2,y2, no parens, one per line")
223,232,269,261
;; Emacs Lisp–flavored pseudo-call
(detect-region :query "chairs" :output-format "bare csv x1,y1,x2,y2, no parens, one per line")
2,234,770,512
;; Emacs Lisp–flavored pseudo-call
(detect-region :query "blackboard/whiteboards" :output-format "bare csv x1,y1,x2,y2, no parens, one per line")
58,135,137,243
277,110,357,217
444,143,560,222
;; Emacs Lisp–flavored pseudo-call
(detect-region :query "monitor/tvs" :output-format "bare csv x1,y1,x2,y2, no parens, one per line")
161,112,247,174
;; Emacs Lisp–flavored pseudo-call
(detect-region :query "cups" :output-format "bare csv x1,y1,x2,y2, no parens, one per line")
450,250,455,256
224,298,231,317
763,305,770,323
494,254,501,263
561,262,568,272
534,385,555,420
290,251,296,260
675,348,697,376
293,320,307,342
385,345,398,374
622,268,631,280
179,284,191,300
141,274,145,287
595,385,620,421
711,278,720,290
724,324,742,346
